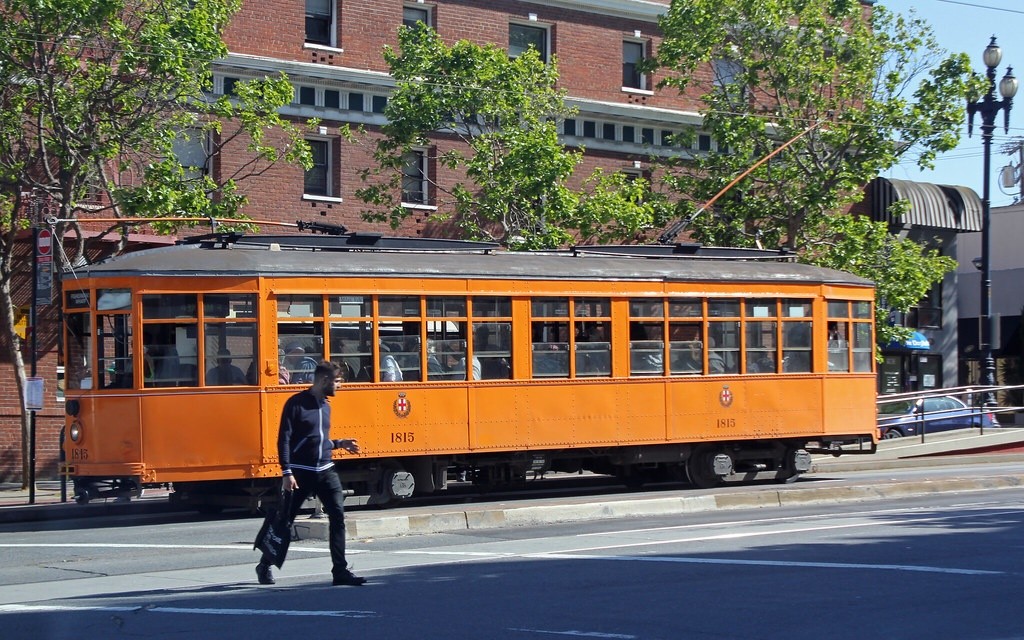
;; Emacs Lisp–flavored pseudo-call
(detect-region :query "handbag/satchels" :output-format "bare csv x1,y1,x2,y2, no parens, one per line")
253,490,294,569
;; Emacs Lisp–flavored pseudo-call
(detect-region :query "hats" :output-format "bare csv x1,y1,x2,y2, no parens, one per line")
104,362,115,372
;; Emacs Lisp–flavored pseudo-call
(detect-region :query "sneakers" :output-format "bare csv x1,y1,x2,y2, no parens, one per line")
256,564,276,584
333,568,366,585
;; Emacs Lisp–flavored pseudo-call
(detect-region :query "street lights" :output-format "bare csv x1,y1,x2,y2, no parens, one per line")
968,33,1017,426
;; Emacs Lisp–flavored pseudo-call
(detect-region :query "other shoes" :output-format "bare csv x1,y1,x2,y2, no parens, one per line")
113,497,131,503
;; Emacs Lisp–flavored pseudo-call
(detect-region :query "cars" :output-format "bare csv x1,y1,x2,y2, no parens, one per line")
876,388,1003,439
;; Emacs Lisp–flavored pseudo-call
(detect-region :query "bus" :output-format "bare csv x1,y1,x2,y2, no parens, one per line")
54,114,880,514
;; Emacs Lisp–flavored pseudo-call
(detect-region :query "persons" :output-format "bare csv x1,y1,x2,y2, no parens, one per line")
549,315,846,374
255,362,368,585
207,324,513,384
122,339,156,389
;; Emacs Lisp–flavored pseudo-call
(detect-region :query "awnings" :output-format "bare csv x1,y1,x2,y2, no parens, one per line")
871,176,984,233
877,327,931,351
